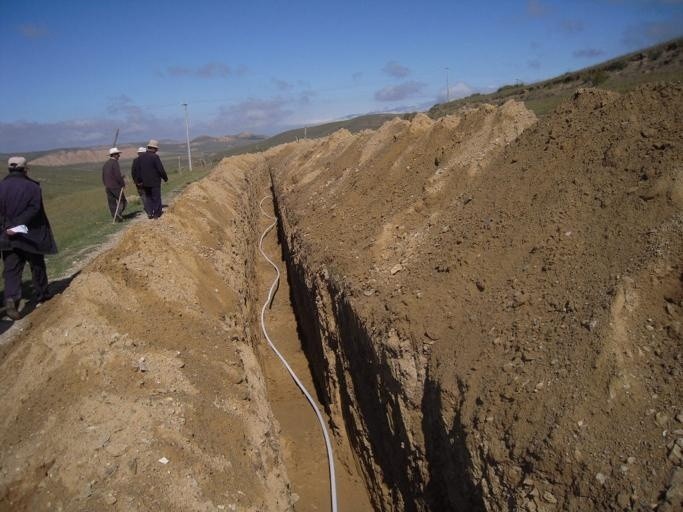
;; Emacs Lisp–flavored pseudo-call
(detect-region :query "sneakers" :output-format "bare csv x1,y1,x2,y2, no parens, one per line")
32,292,53,304
144,208,164,219
6,297,22,320
112,212,124,222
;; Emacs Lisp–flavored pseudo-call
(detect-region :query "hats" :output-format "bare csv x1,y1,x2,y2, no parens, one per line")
137,146,146,154
147,139,161,149
7,157,30,169
105,147,123,155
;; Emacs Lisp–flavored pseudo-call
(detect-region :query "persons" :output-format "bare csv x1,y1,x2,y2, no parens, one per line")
0,156,58,320
132,147,151,215
103,148,128,222
138,140,168,219
